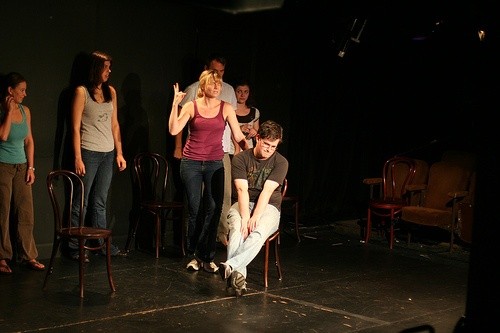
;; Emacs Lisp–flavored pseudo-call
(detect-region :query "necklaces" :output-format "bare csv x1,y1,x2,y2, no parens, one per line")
95,91,103,98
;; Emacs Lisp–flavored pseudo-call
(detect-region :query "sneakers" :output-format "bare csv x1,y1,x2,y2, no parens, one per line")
201,260,219,272
227,271,245,296
219,261,233,280
186,259,199,271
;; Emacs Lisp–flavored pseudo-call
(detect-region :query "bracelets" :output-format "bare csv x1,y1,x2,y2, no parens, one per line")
28,167,36,170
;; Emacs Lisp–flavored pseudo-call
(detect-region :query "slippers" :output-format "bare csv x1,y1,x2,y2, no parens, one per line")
28,259,45,271
0,260,13,274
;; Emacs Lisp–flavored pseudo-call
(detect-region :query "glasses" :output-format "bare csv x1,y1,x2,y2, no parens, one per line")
260,139,277,151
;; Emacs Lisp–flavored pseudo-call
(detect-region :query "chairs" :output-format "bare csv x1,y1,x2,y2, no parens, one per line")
363,157,476,255
43,170,116,298
126,152,185,259
262,179,301,288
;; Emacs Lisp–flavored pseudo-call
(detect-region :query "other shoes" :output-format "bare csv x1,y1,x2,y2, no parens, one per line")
75,254,90,263
119,252,128,257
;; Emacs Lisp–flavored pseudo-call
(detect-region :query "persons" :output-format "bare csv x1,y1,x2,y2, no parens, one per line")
173,58,236,247
217,121,289,298
228,83,260,161
64,52,131,262
0,72,46,274
168,68,251,273
